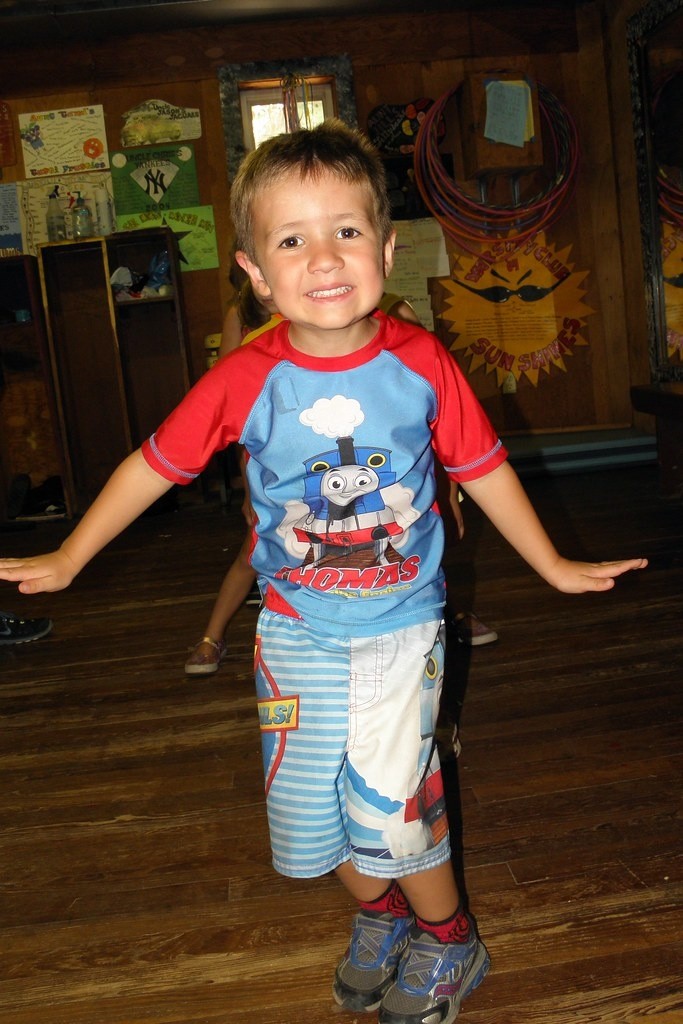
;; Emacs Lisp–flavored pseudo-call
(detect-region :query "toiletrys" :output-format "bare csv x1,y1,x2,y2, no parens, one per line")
70,192,91,237
46,186,66,241
95,187,112,234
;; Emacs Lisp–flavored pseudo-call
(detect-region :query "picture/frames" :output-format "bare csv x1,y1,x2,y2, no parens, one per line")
621,0,683,387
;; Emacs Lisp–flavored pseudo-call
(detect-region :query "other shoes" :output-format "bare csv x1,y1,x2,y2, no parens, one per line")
246,580,262,606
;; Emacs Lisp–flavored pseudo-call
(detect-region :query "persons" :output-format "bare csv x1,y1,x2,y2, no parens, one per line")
175,223,499,676
0,608,52,644
0,120,649,1024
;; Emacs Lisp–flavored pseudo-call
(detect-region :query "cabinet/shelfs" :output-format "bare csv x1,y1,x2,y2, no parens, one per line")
1,251,76,525
32,227,207,512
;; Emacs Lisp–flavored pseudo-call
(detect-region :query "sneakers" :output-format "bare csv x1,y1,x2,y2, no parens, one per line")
331,906,416,1014
0,610,55,648
379,924,492,1024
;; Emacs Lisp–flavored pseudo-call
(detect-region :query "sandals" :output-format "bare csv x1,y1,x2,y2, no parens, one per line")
184,635,230,674
447,610,499,645
